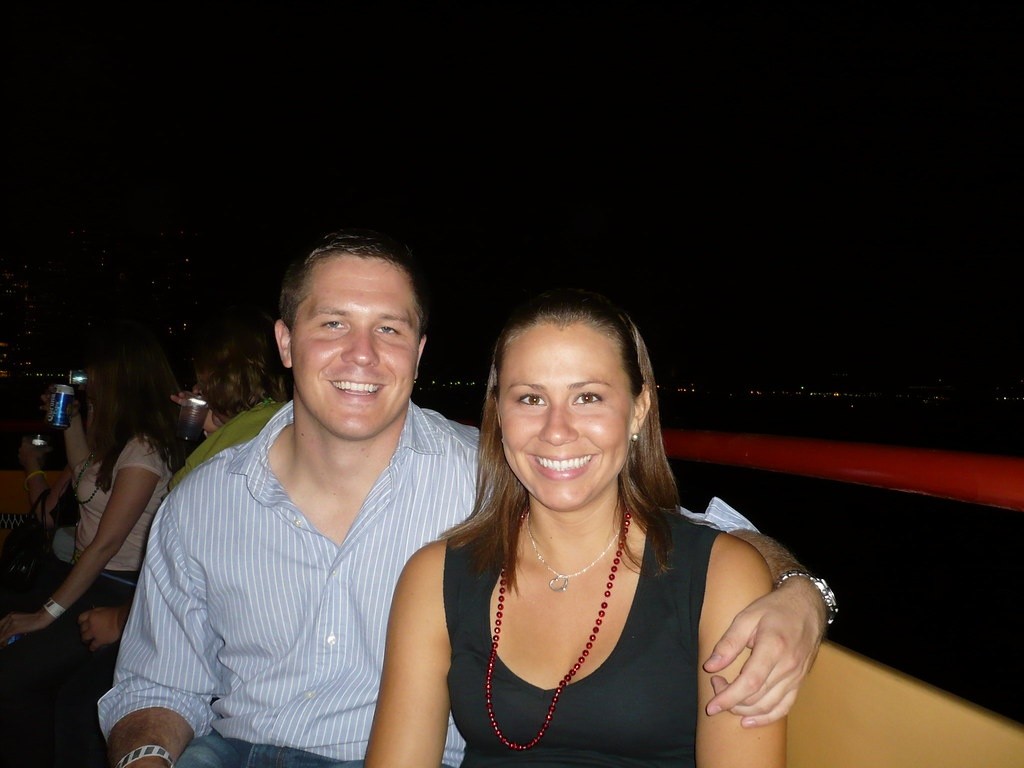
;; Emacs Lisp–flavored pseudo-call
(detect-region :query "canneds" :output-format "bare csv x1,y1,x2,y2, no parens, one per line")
47,385,74,429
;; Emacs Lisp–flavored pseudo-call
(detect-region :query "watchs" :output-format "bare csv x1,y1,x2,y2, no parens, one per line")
775,570,839,625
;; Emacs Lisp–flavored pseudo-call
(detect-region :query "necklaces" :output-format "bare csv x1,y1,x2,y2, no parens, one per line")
73,448,99,504
484,505,632,750
526,512,620,593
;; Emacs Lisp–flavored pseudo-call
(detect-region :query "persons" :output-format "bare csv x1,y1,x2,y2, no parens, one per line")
97,231,828,768
364,287,787,768
0,304,287,768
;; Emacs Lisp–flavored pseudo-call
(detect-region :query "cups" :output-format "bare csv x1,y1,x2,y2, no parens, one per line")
26,434,49,467
176,391,210,442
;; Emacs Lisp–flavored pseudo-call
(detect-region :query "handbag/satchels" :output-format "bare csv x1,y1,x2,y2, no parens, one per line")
0,488,51,598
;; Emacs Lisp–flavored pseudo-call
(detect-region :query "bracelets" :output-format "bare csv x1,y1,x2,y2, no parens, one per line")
116,745,174,768
24,470,46,492
